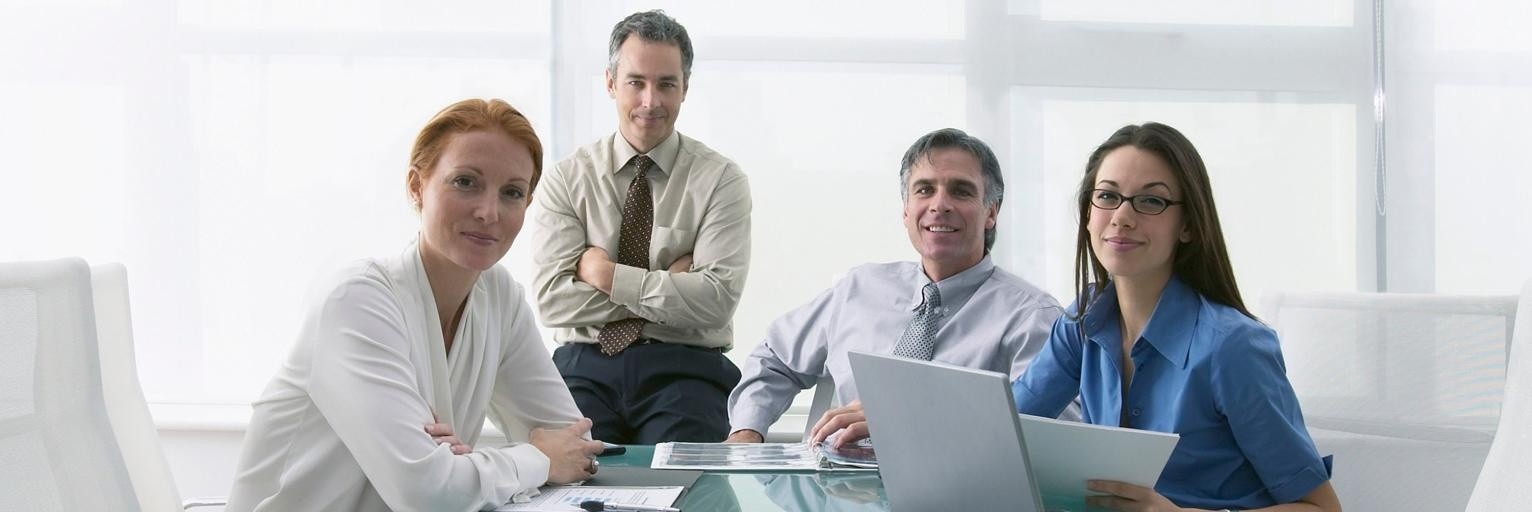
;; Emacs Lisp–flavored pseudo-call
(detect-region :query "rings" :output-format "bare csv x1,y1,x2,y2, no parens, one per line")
588,456,599,474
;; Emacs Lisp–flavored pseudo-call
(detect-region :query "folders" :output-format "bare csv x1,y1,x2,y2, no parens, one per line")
577,499,680,512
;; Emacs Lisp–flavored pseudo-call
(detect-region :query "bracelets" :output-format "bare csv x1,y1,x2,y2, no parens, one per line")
1223,507,1230,512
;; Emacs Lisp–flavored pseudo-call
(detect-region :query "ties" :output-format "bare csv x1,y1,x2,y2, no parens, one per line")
598,154,653,356
890,282,941,363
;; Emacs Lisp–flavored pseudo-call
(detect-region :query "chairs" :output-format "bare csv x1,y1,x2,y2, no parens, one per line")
89,263,229,512
1275,291,1520,512
0,260,141,511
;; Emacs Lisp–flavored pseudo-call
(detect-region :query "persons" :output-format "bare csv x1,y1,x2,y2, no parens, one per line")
228,99,603,511
1011,121,1344,512
754,472,889,512
728,129,1085,445
531,9,741,441
598,439,743,512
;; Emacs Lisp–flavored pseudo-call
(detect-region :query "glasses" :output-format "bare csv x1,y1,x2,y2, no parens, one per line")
1086,187,1188,216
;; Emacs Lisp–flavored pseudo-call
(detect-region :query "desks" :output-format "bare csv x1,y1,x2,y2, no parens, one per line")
467,445,1119,512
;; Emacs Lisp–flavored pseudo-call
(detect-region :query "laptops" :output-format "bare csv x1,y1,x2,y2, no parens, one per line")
847,347,1045,510
